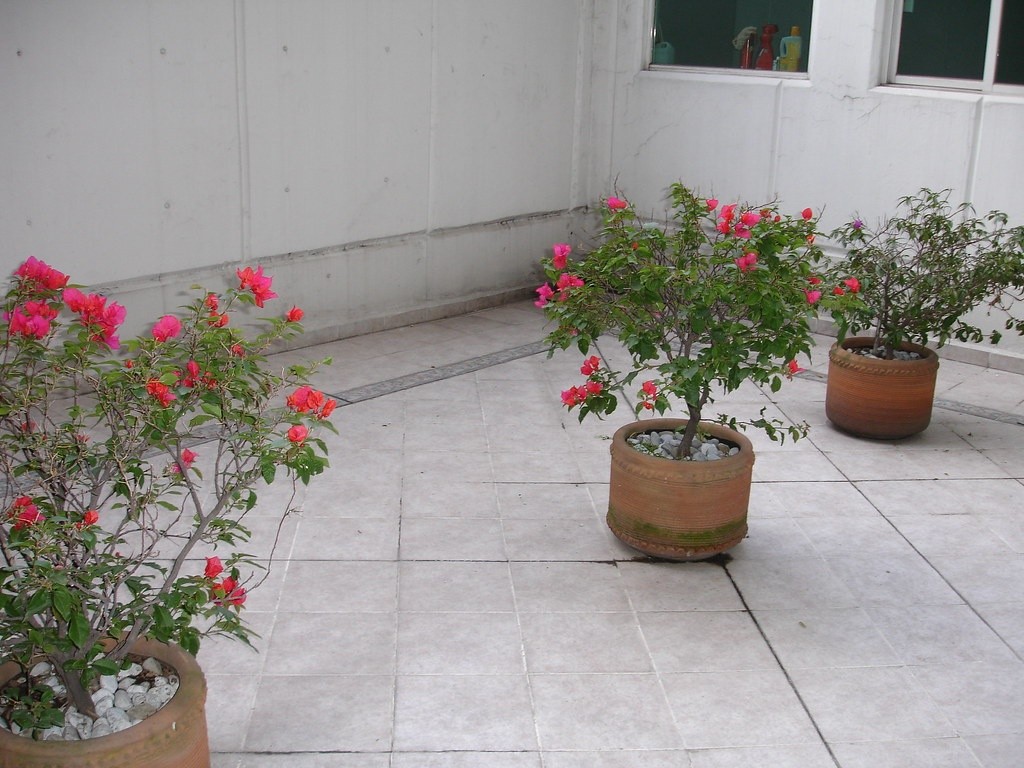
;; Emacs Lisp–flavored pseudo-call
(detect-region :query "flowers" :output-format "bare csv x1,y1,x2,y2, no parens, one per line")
533,171,870,455
0,257,339,731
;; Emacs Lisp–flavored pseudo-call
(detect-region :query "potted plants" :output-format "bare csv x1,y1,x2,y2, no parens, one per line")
826,188,1024,439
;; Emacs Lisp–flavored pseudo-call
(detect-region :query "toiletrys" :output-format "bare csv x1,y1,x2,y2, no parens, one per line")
741,31,761,70
778,25,803,71
754,24,780,70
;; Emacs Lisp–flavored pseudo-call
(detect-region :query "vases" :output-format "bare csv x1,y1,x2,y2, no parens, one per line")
0,632,211,768
607,419,756,562
